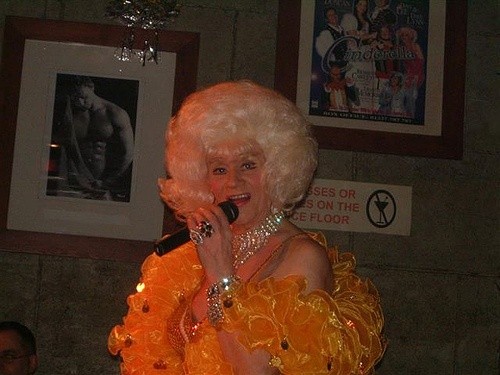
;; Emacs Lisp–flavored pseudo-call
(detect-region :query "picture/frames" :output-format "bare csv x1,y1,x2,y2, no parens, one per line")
0,14,202,266
274,0,469,159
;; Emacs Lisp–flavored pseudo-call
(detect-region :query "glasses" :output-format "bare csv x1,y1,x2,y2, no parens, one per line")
0,354,32,364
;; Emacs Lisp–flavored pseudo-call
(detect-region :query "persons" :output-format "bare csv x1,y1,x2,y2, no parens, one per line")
62,77,134,201
316,0,424,117
0,321,37,375
109,79,389,375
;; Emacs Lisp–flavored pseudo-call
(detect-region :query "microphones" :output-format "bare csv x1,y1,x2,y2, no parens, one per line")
152,200,240,257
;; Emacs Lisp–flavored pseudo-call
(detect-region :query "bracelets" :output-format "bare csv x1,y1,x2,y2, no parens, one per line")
206,274,241,331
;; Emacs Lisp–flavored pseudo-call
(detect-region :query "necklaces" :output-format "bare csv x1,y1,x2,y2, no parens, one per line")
232,211,284,274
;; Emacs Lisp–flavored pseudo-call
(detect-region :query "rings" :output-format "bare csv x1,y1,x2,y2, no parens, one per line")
199,221,214,238
190,228,204,244
